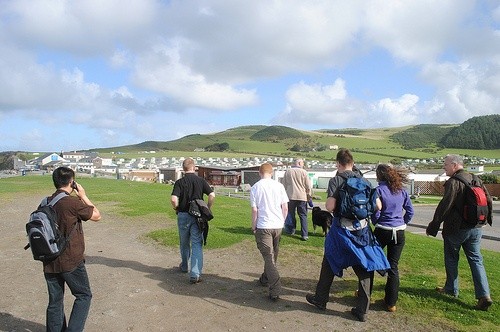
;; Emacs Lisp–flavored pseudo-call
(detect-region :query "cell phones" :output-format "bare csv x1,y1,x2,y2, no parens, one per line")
71,180,79,192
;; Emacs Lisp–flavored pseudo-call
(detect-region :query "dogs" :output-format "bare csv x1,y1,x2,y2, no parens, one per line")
311,206,334,238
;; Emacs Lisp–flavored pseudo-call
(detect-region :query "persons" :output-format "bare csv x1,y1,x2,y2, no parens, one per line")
284,158,312,240
417,186,420,197
306,149,382,322
355,164,414,312
171,158,215,282
250,163,290,301
426,155,493,311
38,166,101,332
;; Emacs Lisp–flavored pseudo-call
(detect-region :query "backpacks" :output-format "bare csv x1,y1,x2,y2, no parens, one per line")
451,173,488,227
335,174,373,217
26,192,69,261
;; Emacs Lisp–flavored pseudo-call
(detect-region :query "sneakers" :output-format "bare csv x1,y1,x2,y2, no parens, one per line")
306,293,326,309
351,308,367,322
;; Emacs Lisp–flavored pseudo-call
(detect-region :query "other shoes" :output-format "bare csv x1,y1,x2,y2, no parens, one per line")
191,276,202,282
284,228,295,234
436,286,445,293
475,297,493,310
302,235,309,241
180,264,189,272
260,277,268,285
383,300,397,312
356,290,376,303
270,291,279,298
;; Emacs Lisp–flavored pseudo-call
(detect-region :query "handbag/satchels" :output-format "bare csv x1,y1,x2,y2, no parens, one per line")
188,202,202,217
